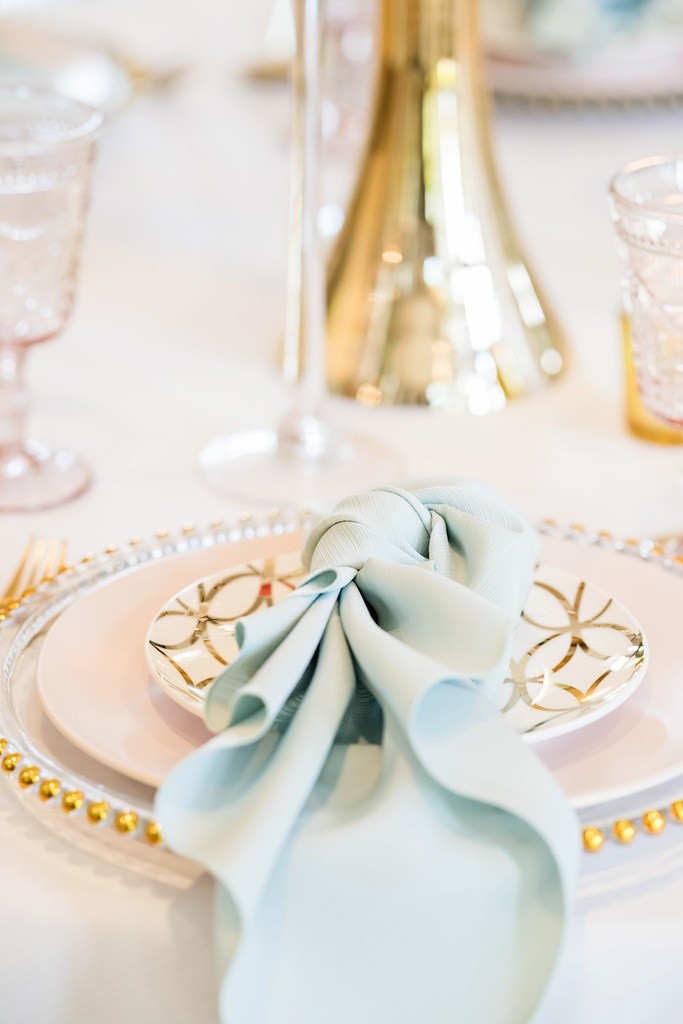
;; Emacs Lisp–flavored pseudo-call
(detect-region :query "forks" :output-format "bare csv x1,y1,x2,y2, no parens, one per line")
0,535,68,607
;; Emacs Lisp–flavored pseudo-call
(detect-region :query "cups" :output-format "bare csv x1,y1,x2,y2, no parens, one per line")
611,151,683,424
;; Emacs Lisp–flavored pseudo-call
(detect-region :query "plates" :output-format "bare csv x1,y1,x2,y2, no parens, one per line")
0,507,682,891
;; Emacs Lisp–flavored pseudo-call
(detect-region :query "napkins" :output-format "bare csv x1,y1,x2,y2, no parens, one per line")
153,478,580,1024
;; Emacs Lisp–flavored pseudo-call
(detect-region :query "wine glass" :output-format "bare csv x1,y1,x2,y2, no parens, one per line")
1,81,99,515
195,0,410,503
